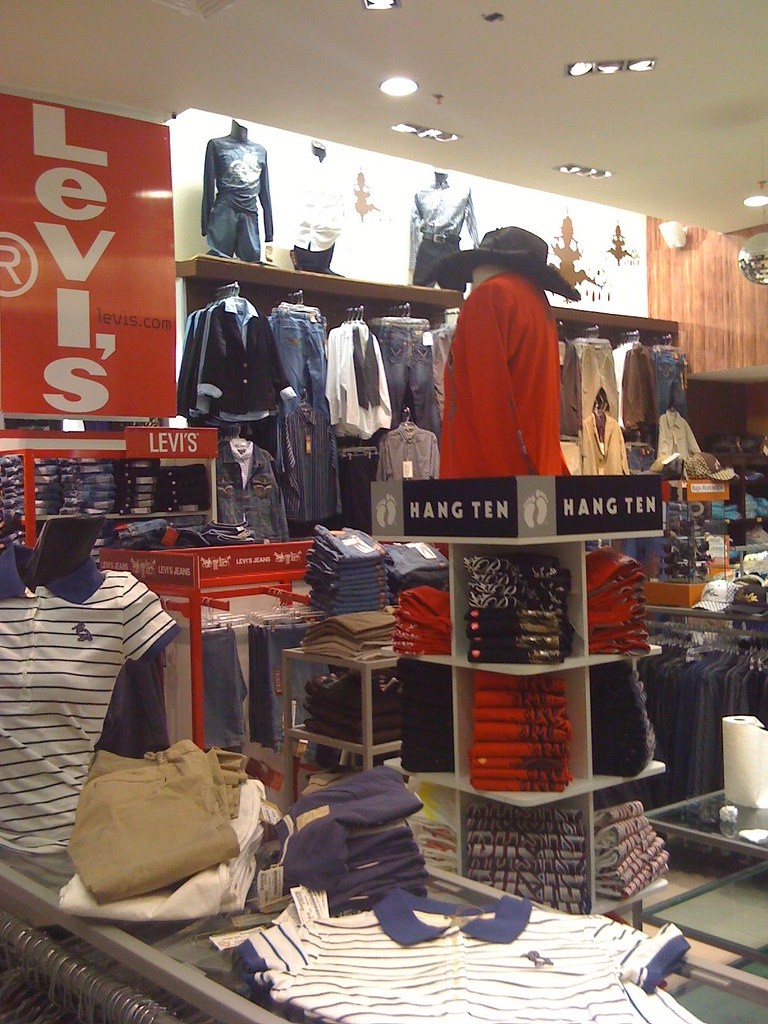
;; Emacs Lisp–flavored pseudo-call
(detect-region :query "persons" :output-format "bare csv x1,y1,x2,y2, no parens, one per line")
0,514,182,856
436,227,582,478
409,171,480,293
201,119,274,263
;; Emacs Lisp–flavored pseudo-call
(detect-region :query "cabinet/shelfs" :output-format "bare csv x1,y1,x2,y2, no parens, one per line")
0,446,218,572
282,645,399,817
394,531,672,922
0,849,768,1024
644,566,734,631
624,452,768,583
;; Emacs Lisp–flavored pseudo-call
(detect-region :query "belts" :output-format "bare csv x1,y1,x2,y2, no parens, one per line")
423,232,460,244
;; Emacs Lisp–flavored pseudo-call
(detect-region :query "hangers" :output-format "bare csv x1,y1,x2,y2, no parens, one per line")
644,606,768,684
200,585,327,637
190,281,692,457
0,905,225,1024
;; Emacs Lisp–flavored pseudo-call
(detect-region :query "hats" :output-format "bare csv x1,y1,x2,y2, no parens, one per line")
437,226,581,302
726,582,767,614
691,579,737,613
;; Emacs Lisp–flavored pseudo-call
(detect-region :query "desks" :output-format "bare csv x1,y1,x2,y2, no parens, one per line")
631,788,768,1024
